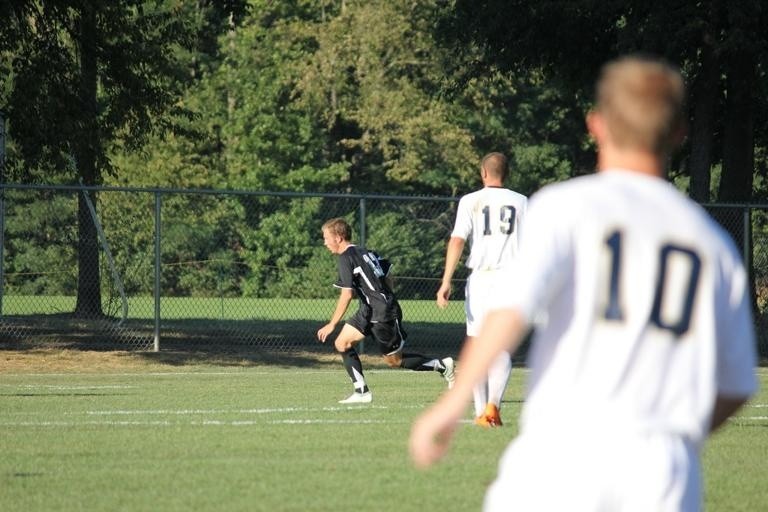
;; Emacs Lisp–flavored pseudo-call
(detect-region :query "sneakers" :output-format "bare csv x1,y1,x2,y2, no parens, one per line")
474,416,488,426
441,357,455,389
485,404,502,426
338,391,373,404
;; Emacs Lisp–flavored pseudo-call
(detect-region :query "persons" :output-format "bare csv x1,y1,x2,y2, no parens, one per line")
407,50,759,512
314,217,458,407
436,151,530,427
